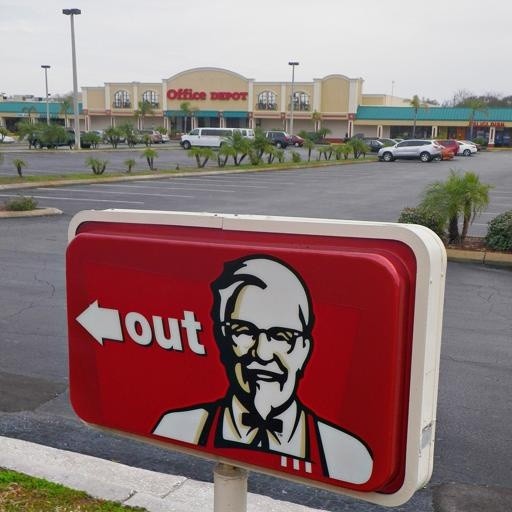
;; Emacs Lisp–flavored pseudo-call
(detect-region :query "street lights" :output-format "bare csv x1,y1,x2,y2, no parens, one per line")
289,62,299,137
41,65,50,125
63,8,81,149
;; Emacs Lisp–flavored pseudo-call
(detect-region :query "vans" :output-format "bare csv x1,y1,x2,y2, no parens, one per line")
179,126,240,149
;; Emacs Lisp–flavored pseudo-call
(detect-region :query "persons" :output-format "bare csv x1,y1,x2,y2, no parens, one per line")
150,254,372,488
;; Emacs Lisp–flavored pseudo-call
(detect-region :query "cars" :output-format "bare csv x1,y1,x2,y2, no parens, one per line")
362,135,482,163
240,128,305,149
0,129,170,149
